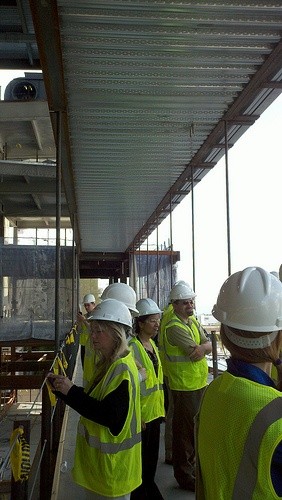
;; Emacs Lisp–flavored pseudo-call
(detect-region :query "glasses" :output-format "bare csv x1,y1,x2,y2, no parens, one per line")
183,302,194,305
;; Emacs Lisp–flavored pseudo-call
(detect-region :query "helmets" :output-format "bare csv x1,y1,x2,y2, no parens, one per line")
85,294,95,304
101,283,140,314
133,298,165,318
168,280,197,299
87,298,132,328
211,266,282,349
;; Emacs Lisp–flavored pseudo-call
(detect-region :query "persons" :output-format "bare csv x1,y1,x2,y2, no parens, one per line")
75,294,95,369
153,279,213,491
49,299,142,500
128,298,164,500
82,283,146,389
193,266,282,500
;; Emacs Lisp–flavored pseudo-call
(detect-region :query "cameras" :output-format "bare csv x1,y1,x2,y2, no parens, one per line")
47,377,56,390
79,312,82,315
